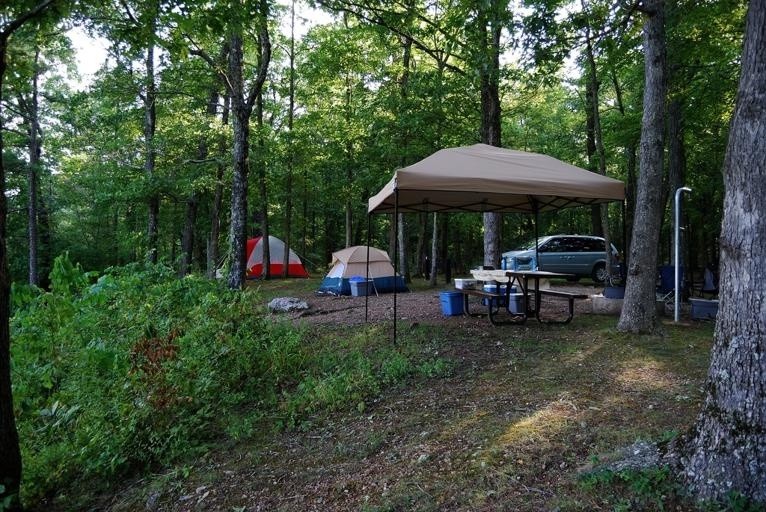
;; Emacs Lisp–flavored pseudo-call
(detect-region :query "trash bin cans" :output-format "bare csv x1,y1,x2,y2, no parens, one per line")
656,265,683,305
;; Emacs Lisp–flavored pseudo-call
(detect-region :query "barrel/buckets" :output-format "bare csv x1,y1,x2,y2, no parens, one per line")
660,264,686,294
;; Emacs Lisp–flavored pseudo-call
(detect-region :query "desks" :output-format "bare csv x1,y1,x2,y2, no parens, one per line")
505,269,576,325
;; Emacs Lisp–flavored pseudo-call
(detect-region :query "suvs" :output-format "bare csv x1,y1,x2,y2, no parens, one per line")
499,234,621,281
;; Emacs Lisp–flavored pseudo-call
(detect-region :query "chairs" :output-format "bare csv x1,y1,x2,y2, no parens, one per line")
655,264,685,313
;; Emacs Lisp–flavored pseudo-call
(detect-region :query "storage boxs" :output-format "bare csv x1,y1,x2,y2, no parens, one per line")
505,293,531,315
605,287,625,299
349,280,374,296
455,279,477,290
690,296,719,320
484,285,517,307
439,291,463,316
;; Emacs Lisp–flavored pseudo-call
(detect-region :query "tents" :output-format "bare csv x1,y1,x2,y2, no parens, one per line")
217,236,308,279
318,245,410,296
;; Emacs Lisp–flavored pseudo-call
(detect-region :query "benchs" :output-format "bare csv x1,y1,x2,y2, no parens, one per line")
455,287,527,328
527,288,588,325
